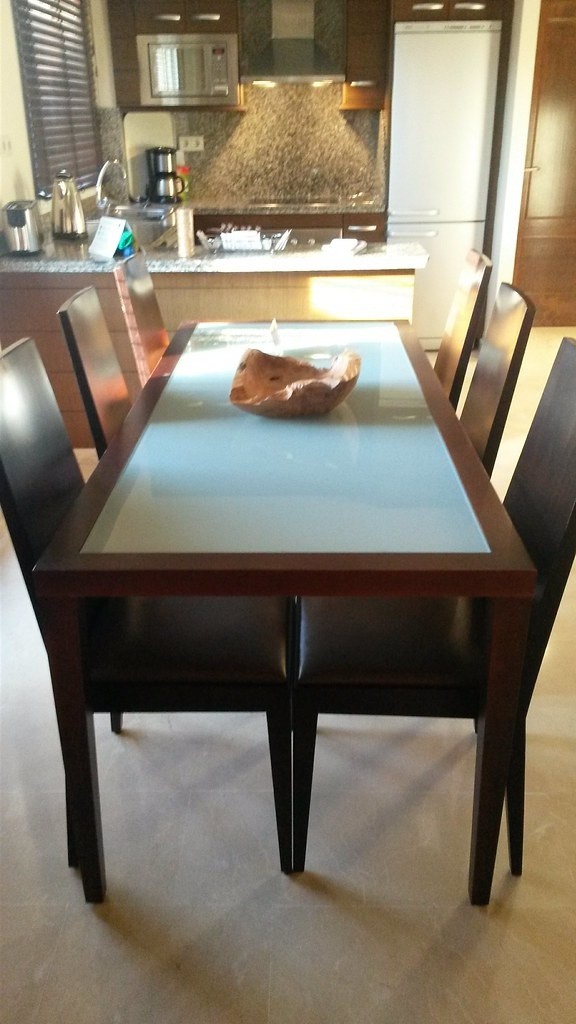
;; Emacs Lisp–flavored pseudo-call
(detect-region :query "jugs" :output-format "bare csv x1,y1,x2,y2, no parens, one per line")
49,168,89,240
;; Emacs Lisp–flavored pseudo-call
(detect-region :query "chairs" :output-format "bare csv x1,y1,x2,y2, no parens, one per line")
458,282,537,480
0,335,298,875
112,251,169,389
293,336,576,875
56,284,134,461
433,248,494,413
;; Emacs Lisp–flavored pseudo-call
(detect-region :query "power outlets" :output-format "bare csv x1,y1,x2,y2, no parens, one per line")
178,136,203,151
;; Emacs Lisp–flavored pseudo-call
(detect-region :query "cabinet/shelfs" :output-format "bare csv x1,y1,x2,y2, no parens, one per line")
108,0,246,110
339,0,391,111
343,214,387,251
391,0,503,22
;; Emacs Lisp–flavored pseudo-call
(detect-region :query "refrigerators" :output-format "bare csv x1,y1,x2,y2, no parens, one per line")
387,19,501,353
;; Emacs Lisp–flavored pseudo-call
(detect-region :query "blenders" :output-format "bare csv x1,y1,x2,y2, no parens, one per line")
148,147,187,202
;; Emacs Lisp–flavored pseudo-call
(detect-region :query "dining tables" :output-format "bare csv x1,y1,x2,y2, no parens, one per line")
30,319,539,908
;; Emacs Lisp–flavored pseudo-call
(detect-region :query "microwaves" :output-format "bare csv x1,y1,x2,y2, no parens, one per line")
135,33,239,106
